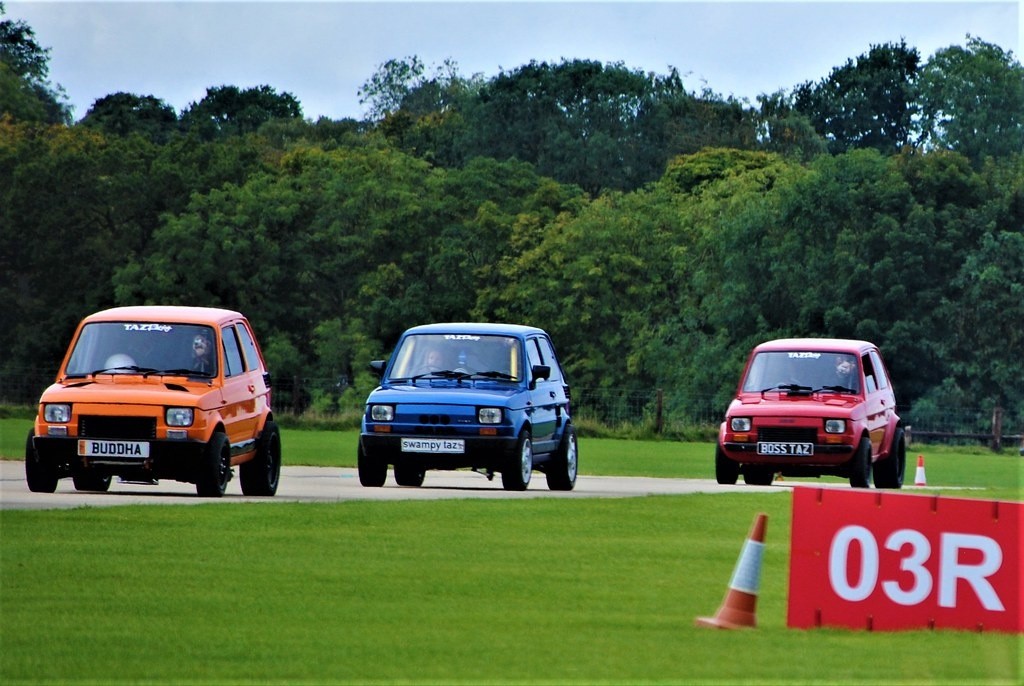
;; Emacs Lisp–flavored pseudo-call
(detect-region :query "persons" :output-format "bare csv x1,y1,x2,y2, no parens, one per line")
421,348,444,374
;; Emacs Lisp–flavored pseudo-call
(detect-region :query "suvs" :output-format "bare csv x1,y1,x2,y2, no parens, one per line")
22,304,283,497
353,319,580,495
713,336,906,492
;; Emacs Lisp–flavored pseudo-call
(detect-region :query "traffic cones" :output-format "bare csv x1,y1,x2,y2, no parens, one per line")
914,454,929,484
696,509,769,631
